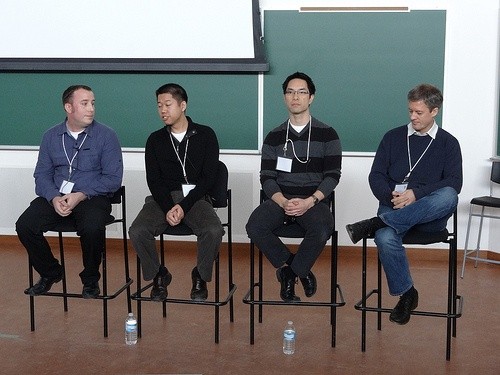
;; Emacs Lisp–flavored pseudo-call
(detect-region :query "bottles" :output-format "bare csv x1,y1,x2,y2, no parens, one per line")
283,321,295,355
124,313,137,345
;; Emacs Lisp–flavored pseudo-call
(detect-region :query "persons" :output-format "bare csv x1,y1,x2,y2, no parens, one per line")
346,84,463,325
128,83,224,301
245,72,342,302
15,86,123,300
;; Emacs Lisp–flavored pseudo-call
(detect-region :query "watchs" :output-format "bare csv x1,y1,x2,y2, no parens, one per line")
312,195,319,204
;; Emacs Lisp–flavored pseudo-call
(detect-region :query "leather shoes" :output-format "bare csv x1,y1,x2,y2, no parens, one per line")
346,219,371,244
276,266,301,302
190,266,208,301
24,274,65,296
150,265,172,302
389,292,418,325
82,281,100,298
293,254,317,297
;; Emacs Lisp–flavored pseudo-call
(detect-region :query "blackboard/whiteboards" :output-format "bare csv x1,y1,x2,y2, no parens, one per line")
0,6,447,157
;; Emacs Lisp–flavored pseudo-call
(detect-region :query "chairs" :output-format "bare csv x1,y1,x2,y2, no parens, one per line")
24,186,133,337
131,161,235,344
242,188,346,347
354,197,463,361
460,162,500,278
